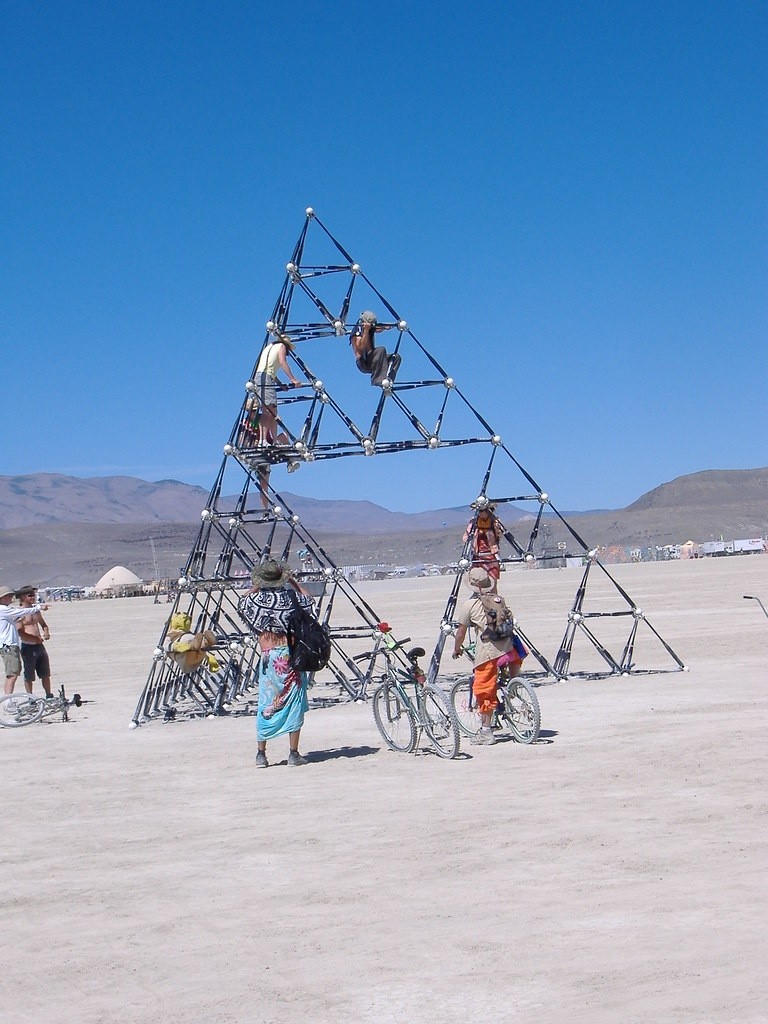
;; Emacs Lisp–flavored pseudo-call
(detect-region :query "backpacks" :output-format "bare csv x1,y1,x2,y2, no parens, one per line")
285,589,331,671
478,593,516,641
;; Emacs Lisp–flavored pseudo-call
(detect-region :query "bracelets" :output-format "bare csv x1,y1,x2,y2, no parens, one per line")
291,378,296,381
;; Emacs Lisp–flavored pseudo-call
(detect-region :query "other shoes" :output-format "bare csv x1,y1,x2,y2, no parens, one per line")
193,631,217,650
287,462,300,473
258,440,272,447
261,513,269,521
4,704,19,715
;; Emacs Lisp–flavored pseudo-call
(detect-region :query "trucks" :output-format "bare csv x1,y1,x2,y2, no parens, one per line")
701,542,724,557
725,539,763,555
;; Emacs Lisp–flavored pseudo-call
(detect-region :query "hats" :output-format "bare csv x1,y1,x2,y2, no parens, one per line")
272,335,295,351
16,586,39,598
241,398,261,410
0,586,15,599
464,567,496,593
470,498,496,509
361,311,377,326
249,559,292,588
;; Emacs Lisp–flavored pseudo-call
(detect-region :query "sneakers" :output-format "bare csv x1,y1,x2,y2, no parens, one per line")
287,751,308,765
256,754,271,768
467,729,497,745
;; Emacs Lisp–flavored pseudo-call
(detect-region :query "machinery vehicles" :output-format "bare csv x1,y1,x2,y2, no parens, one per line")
143,535,168,595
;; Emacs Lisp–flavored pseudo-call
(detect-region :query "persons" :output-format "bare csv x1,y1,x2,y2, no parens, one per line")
238,397,300,521
238,559,309,768
254,335,301,450
349,311,402,386
464,508,502,593
452,567,529,745
15,586,59,707
163,612,219,673
0,586,52,714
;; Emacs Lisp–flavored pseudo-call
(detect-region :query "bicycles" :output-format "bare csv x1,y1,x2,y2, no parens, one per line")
353,637,461,759
0,685,96,727
449,641,541,744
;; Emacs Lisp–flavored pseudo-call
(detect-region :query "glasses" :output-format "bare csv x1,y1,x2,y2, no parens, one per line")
25,593,35,597
